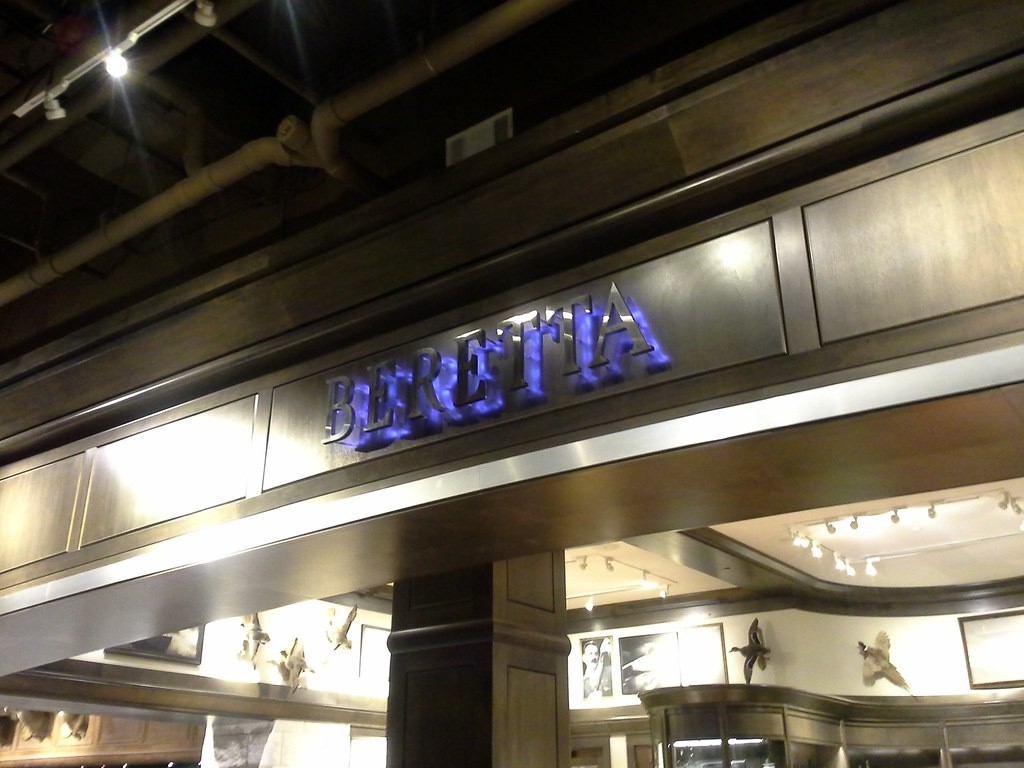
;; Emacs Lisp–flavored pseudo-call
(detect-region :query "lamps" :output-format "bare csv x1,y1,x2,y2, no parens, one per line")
659,584,670,599
998,492,1022,515
928,503,936,518
865,557,880,575
604,557,614,571
850,516,858,529
890,506,900,522
578,555,587,570
826,522,836,533
194,0,217,27
41,91,66,121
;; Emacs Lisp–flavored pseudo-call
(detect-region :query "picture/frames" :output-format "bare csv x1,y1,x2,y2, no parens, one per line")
957,608,1024,690
579,635,615,701
104,625,205,666
618,621,729,695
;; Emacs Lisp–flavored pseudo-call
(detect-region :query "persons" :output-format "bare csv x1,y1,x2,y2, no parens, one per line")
581,634,612,701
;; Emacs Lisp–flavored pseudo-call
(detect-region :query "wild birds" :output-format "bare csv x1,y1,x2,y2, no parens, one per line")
858,630,912,690
240,604,361,695
727,617,772,684
